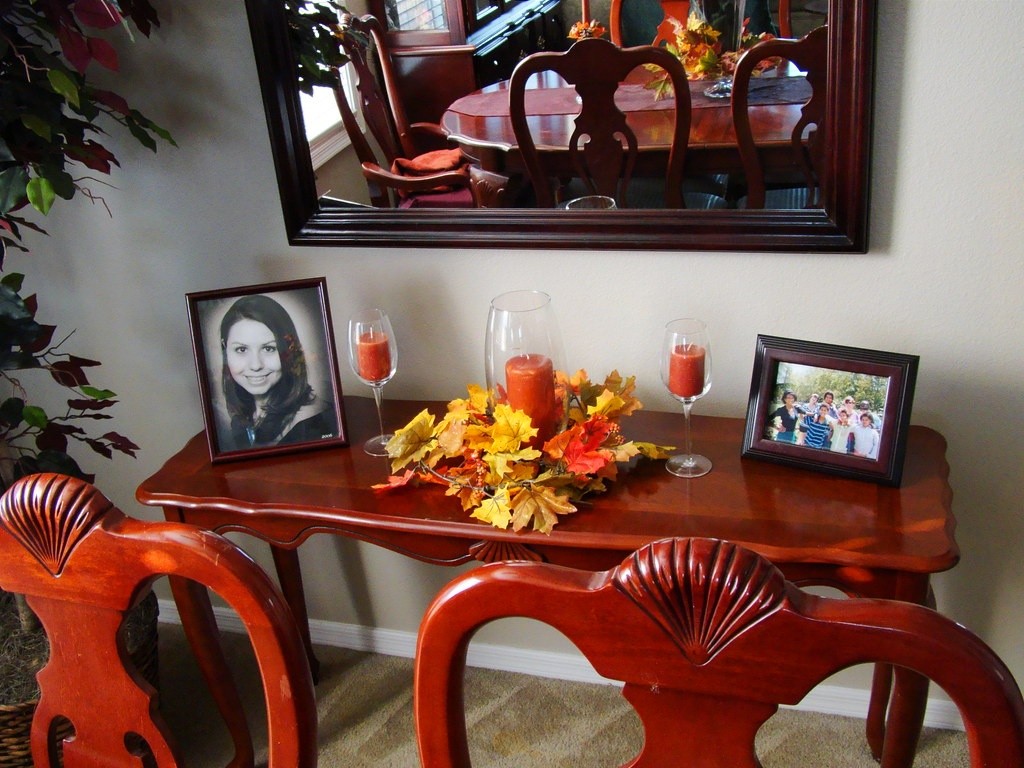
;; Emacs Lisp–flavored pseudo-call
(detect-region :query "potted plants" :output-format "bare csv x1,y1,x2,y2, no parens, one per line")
0,0,182,768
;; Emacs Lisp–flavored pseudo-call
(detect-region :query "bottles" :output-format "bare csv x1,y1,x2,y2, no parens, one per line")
691,0,747,97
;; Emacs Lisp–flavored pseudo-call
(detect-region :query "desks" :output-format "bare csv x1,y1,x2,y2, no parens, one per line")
439,57,816,208
136,396,961,768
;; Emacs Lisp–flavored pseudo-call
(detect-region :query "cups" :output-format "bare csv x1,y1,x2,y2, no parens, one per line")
566,196,618,210
484,289,570,451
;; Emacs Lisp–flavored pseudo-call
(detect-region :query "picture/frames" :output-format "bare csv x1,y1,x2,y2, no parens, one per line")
190,275,351,464
740,332,921,490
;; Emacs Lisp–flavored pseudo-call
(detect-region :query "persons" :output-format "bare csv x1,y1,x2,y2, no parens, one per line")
221,295,338,450
766,358,882,459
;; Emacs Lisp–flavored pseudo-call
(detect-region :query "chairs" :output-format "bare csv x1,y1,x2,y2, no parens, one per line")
0,471,318,768
413,537,1024,768
329,13,525,209
608,0,701,49
511,35,693,214
730,22,829,210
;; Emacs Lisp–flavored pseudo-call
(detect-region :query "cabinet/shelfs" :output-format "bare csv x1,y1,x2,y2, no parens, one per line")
365,0,564,161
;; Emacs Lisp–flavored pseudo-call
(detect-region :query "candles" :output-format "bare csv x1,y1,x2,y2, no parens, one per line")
506,351,558,453
357,324,392,380
670,344,707,399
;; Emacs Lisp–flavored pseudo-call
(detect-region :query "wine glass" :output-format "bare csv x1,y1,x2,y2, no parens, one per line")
348,308,398,457
661,318,713,478
